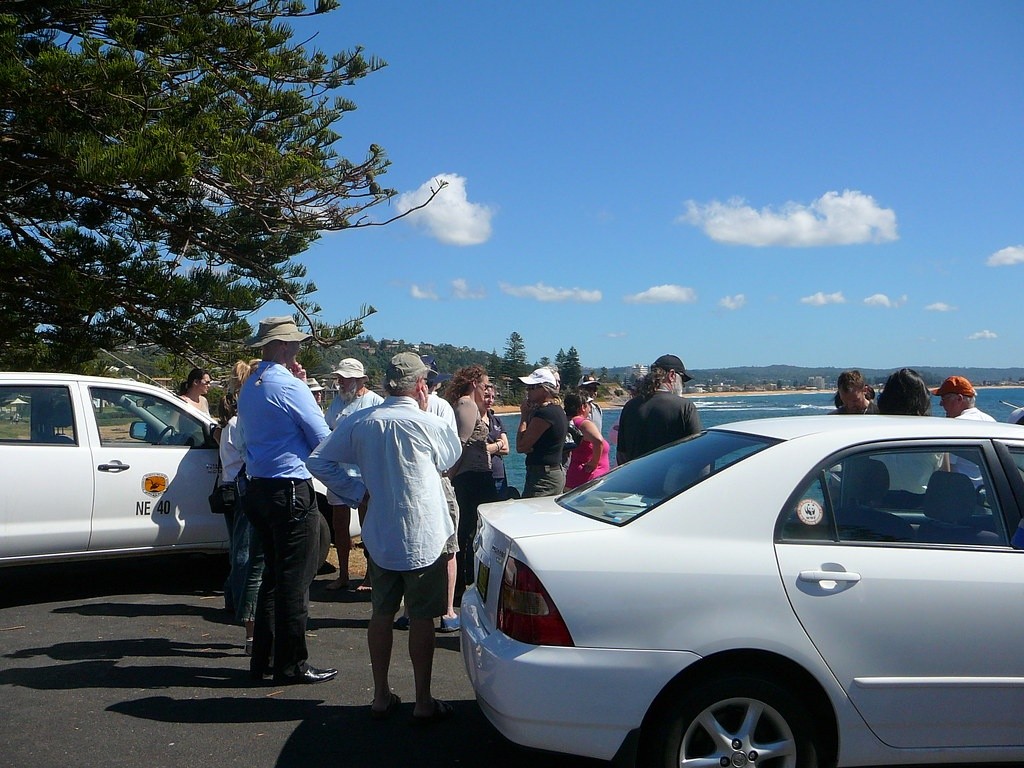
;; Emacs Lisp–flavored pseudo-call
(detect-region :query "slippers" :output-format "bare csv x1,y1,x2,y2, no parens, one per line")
412,696,453,719
370,691,400,712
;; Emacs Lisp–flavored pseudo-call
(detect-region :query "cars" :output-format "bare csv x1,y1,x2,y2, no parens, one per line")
0,373,365,566
458,413,1024,768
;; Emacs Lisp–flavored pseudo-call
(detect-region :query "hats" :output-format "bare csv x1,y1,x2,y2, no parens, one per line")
932,375,975,397
579,375,601,388
651,354,694,382
244,316,313,348
386,353,438,388
517,367,557,387
329,357,369,382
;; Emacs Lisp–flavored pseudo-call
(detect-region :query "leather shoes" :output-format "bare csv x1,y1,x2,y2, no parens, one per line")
272,663,339,684
249,659,275,676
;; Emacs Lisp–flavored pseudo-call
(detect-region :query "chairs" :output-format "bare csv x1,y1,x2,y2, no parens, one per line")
918,470,1004,546
40,399,76,445
840,457,916,543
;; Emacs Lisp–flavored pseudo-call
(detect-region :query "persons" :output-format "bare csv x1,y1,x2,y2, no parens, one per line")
325,358,372,593
306,352,461,727
396,365,460,632
237,315,338,686
563,389,611,493
827,370,881,499
167,368,211,432
443,362,497,607
307,378,324,404
932,376,996,496
578,375,602,434
617,354,710,499
212,359,263,657
868,368,950,509
610,377,644,467
516,367,582,498
476,381,509,501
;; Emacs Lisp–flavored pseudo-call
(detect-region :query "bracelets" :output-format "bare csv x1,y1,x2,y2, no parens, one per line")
497,439,504,449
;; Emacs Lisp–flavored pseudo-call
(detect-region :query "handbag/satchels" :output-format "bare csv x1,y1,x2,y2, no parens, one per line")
208,484,238,513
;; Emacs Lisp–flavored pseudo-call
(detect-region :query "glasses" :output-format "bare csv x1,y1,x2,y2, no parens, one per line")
940,394,956,406
478,380,488,392
199,379,210,386
582,387,597,393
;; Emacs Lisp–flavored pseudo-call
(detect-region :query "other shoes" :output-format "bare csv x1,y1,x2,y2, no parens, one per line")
393,616,410,629
244,638,253,655
355,579,373,593
326,578,349,588
441,614,461,632
